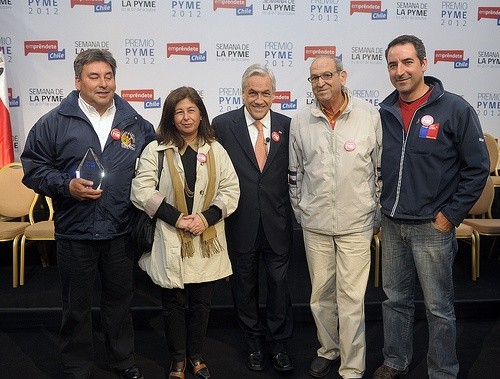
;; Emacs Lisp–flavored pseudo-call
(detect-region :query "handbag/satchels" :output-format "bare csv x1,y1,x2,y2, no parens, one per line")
127,204,158,251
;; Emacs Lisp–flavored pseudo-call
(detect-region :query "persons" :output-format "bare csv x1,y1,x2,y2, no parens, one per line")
374,35,490,379
288,55,383,379
130,87,241,379
211,63,295,379
20,49,159,379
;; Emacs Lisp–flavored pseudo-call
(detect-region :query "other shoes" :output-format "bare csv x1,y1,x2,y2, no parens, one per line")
371,364,410,379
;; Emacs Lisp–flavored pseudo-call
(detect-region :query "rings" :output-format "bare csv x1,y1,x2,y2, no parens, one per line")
196,230,198,233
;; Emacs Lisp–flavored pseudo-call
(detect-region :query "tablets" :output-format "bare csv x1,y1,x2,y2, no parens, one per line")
75,148,104,190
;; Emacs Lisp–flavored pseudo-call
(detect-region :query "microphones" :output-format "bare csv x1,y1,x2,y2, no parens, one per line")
264,137,270,145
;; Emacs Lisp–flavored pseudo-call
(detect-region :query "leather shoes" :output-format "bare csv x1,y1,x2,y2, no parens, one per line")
169,358,186,379
271,350,296,373
247,351,266,371
308,356,331,375
121,366,144,379
187,355,213,379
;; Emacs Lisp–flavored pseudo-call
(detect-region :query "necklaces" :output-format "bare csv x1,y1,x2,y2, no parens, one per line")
185,180,194,197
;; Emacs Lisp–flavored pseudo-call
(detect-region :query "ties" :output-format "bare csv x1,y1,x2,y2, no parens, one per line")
254,117,268,175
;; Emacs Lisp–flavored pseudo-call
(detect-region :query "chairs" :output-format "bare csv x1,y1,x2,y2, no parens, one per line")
0,133,500,288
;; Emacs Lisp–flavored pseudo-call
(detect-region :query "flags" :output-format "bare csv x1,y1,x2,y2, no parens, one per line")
0,47,15,169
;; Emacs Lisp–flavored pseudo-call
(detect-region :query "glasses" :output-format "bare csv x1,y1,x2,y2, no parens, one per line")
308,70,339,82
